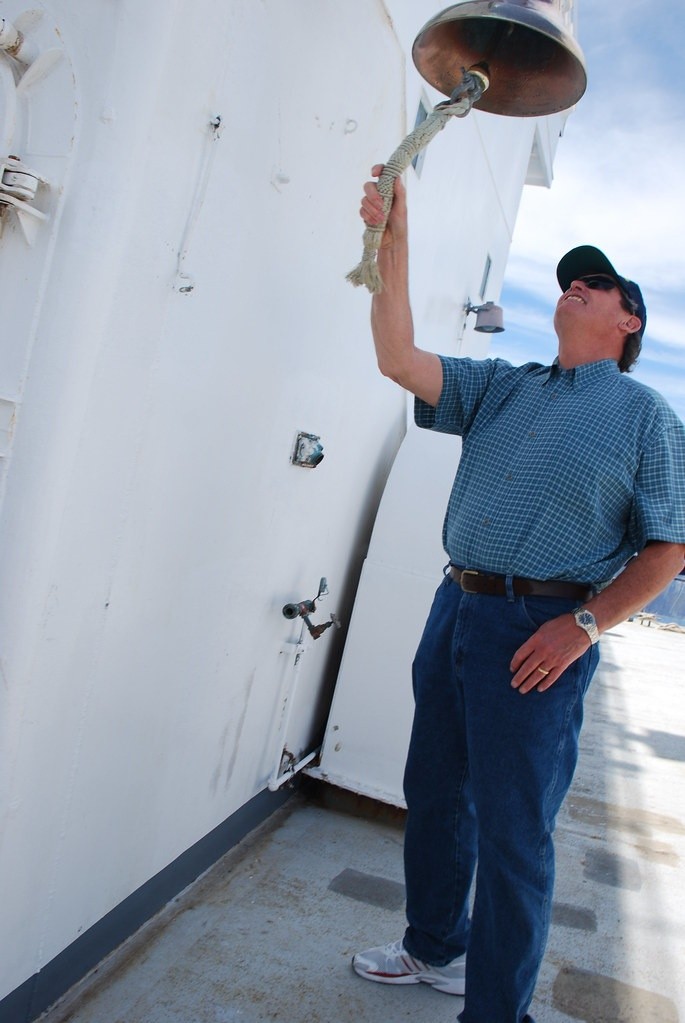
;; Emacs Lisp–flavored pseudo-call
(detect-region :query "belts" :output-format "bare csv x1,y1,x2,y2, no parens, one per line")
448,564,595,603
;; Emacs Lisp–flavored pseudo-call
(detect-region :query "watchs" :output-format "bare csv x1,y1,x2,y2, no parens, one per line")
571,606,600,645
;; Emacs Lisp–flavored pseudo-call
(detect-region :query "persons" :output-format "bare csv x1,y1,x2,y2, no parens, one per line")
342,163,683,1023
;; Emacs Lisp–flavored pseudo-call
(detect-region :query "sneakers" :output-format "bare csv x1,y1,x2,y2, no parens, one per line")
351,936,466,995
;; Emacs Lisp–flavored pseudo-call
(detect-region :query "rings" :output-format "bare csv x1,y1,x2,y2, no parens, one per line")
538,666,550,674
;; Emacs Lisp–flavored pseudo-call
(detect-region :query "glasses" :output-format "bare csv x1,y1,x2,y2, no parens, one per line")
573,275,636,315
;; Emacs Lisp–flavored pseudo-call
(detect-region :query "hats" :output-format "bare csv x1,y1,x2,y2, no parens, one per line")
556,245,647,335
